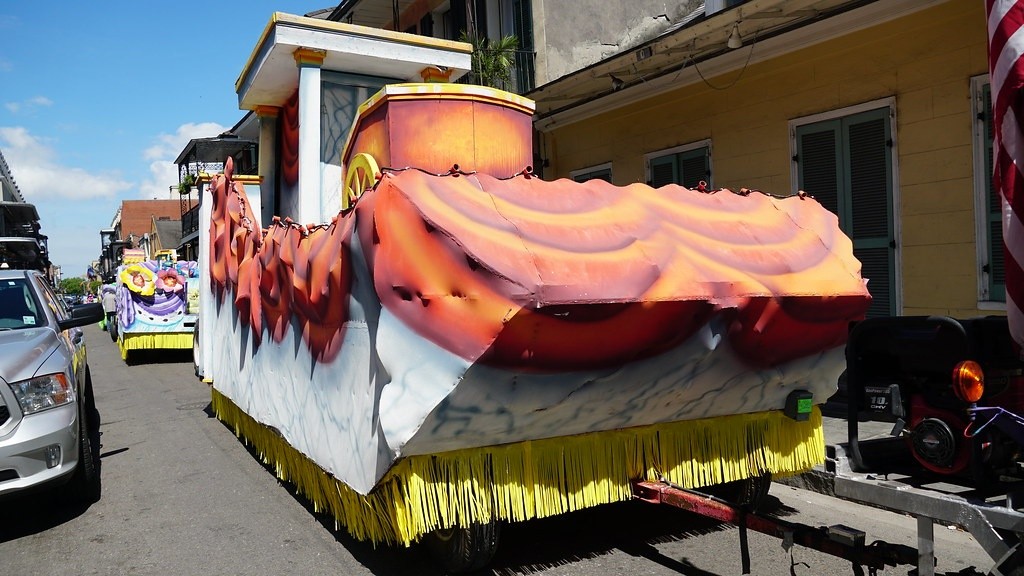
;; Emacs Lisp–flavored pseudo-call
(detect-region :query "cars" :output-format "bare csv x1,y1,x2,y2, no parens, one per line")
0,268,106,509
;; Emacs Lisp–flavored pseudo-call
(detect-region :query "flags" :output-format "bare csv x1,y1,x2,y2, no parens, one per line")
988,0,1024,350
